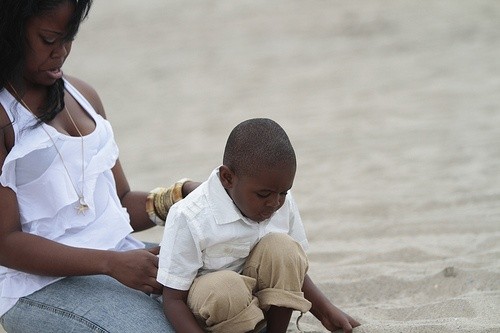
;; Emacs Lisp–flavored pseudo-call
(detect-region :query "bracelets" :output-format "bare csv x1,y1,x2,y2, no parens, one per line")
145,177,192,226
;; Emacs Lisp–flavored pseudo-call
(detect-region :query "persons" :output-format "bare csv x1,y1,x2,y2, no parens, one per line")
0,0,202,333
155,118,361,333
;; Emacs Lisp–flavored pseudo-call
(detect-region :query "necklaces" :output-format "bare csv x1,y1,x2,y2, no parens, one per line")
9,83,90,216
